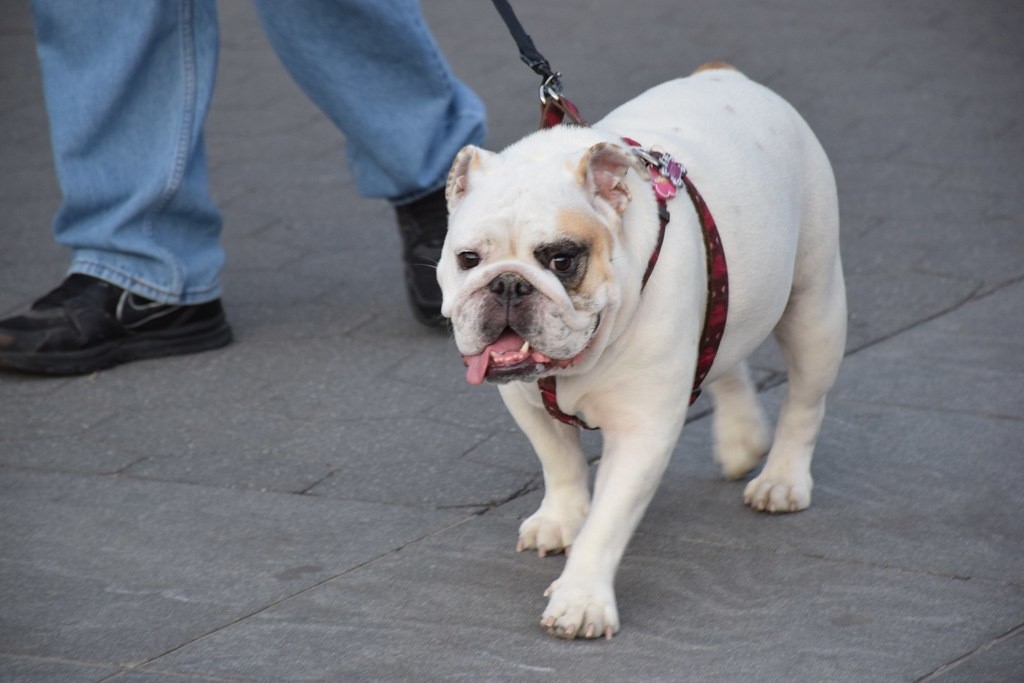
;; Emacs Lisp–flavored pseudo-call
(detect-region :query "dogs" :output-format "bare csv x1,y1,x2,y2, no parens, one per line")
434,62,847,642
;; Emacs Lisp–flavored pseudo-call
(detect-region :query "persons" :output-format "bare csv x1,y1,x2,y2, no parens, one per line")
0,0,487,378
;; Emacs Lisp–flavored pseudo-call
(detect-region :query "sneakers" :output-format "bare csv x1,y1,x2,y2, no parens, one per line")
395,186,456,329
0,271,231,375
